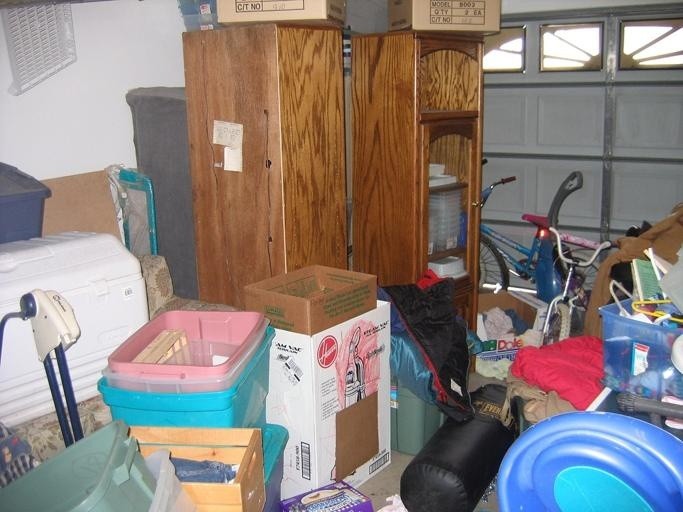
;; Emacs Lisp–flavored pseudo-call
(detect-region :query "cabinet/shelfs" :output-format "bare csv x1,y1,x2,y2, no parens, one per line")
350,31,485,334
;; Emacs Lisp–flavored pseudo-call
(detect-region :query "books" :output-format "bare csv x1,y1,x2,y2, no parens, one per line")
631,246,674,306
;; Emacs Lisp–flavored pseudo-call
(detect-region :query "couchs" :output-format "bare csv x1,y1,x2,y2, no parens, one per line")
1,396,115,465
140,253,238,319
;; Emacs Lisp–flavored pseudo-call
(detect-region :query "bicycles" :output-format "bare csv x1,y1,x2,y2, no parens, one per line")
479,159,612,309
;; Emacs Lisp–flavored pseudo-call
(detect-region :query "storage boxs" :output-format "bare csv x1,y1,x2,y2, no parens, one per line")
215,0,346,28
276,482,374,512
1,161,51,245
2,420,157,512
243,263,377,336
598,297,683,402
95,310,289,512
39,165,157,260
386,1,501,35
0,231,151,428
266,299,392,501
